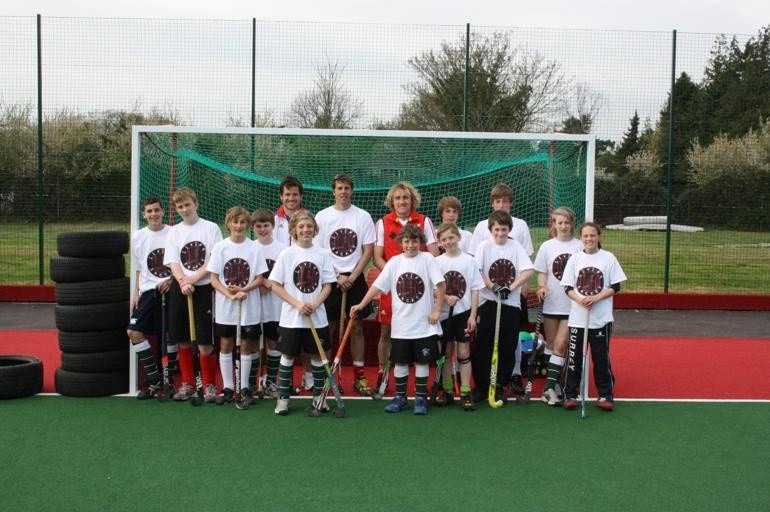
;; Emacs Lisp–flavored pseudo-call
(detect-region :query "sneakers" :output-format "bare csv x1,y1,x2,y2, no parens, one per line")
413,398,428,413
240,388,257,404
384,398,409,411
352,375,375,396
171,383,195,402
274,397,289,414
250,386,258,399
596,395,612,410
434,389,453,405
541,388,562,407
215,388,234,404
154,384,176,397
376,375,389,394
302,374,314,391
555,383,562,396
461,394,475,409
138,381,163,400
203,384,218,403
265,378,277,397
312,395,330,413
565,399,578,409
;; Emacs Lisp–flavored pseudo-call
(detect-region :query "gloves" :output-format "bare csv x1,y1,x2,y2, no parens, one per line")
491,281,512,300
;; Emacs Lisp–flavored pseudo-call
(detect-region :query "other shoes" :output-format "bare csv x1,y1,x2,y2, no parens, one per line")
509,375,526,395
474,384,489,404
493,384,507,403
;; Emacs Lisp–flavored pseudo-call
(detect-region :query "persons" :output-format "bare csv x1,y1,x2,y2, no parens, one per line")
432,196,473,392
433,222,486,411
349,224,446,415
373,183,441,394
249,208,288,400
474,210,535,402
268,211,337,415
532,208,584,406
467,183,534,395
206,204,270,405
311,173,377,396
273,175,315,391
162,187,223,403
127,198,179,400
560,222,627,411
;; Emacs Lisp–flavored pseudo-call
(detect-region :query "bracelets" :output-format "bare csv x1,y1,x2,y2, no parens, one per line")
335,271,340,280
535,285,546,290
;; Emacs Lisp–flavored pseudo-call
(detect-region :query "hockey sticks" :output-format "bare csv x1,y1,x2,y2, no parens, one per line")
155,291,172,404
490,294,503,409
292,345,306,396
337,288,347,395
258,322,263,400
580,306,590,419
517,292,545,405
187,295,205,406
428,305,455,405
234,299,249,410
306,309,357,418
371,359,392,400
